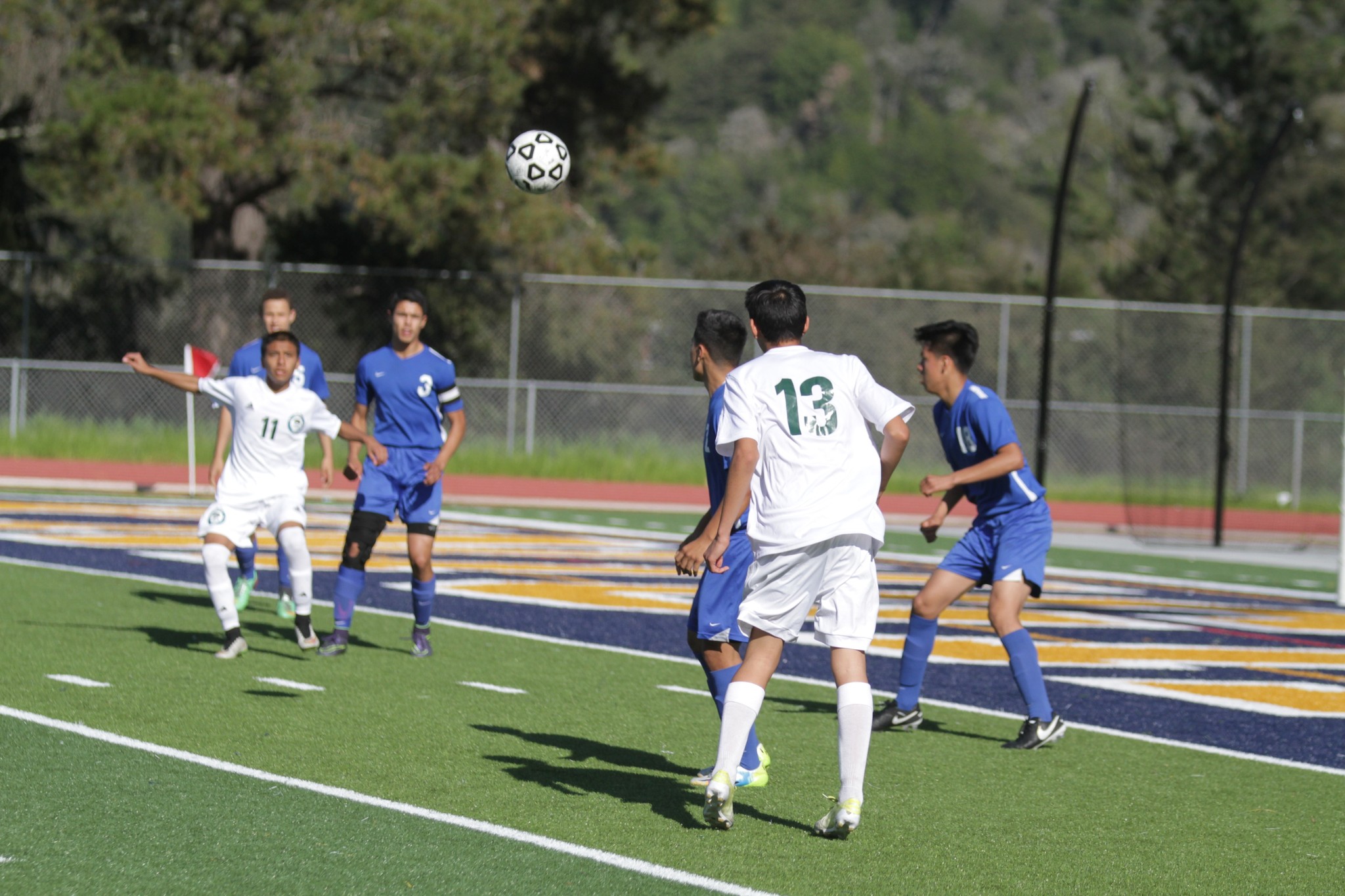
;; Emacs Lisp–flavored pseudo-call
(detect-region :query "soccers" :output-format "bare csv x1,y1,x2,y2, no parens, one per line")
504,129,572,195
1276,491,1292,509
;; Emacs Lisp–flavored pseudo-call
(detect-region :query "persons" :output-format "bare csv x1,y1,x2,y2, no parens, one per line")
700,280,917,840
207,287,335,618
676,308,773,788
869,317,1071,752
119,330,390,664
316,288,465,656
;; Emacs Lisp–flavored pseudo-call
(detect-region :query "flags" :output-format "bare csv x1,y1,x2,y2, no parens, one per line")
184,342,224,388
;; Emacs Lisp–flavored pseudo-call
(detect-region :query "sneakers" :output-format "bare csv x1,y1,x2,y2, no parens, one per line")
215,635,249,660
295,621,321,650
698,743,771,778
813,793,862,839
872,699,924,731
691,762,769,788
276,586,296,620
316,633,351,656
702,770,735,830
409,620,432,658
233,570,258,612
1002,711,1067,750
